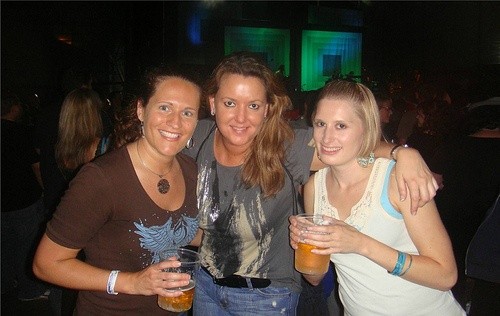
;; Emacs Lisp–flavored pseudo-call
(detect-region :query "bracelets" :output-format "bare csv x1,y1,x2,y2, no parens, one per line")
107,270,121,294
400,254,412,277
387,251,406,276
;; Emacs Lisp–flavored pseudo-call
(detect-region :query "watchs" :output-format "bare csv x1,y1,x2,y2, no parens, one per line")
389,142,408,162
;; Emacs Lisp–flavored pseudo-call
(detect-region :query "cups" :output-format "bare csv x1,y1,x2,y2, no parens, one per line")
293,213,337,276
157,249,202,312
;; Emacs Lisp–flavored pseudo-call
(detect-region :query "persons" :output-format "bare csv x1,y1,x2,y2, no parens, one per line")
0,53,500,316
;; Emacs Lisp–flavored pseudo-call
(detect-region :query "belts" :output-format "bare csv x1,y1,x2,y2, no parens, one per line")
201,264,272,288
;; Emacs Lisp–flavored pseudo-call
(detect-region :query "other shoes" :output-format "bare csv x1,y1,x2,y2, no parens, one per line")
17,288,51,302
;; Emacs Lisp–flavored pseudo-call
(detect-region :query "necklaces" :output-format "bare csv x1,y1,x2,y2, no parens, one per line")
136,137,171,194
